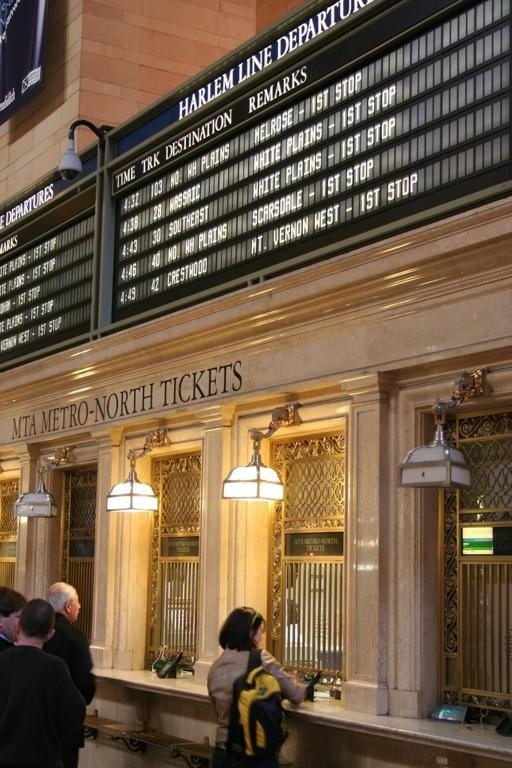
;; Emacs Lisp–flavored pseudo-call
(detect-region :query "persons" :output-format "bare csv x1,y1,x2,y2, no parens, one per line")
0,597,88,768
206,605,309,768
42,581,96,705
0,585,28,652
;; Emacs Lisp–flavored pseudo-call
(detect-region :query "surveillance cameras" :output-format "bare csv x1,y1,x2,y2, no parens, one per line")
59,152,83,182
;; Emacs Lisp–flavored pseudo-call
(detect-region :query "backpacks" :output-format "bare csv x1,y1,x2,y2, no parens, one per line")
226,648,290,759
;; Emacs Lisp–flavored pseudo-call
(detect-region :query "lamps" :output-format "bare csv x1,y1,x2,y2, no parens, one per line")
104,427,168,512
221,399,306,503
392,367,491,489
13,441,76,517
58,117,105,182
